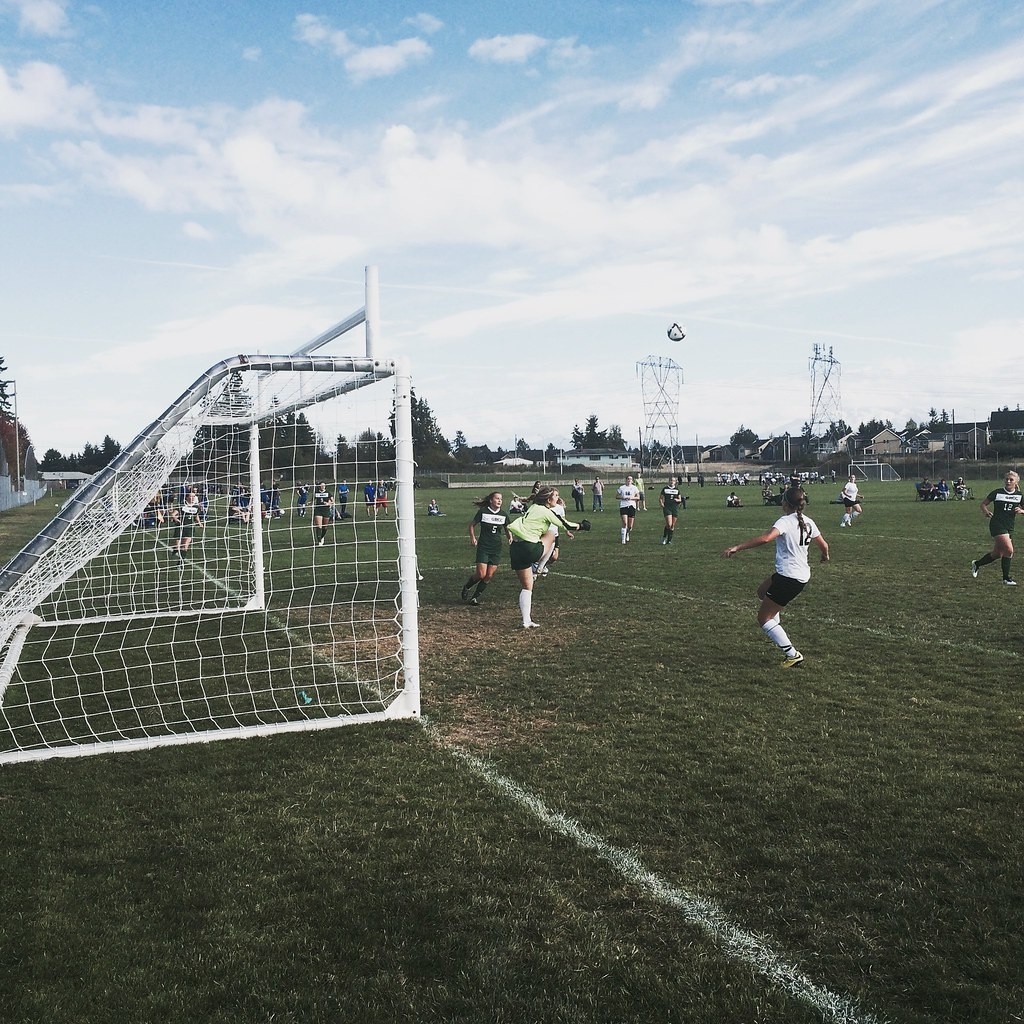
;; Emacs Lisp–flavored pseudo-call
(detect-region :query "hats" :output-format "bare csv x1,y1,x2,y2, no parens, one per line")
959,477,962,480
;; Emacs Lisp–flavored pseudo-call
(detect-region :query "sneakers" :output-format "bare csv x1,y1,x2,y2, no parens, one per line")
972,560,978,578
841,523,845,527
662,537,672,545
622,533,630,544
523,622,541,628
781,650,804,668
462,585,468,599
533,561,549,581
847,519,852,527
469,598,479,606
1004,580,1017,586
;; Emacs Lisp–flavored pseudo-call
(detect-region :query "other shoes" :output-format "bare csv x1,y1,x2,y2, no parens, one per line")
601,510,603,511
274,517,280,519
593,510,596,512
319,537,324,545
962,497,966,501
637,508,640,511
643,508,647,510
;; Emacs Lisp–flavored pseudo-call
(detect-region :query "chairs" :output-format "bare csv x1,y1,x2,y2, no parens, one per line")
951,481,970,500
762,487,808,506
915,483,943,501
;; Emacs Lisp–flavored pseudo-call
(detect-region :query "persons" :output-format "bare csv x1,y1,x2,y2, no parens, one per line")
919,476,969,501
591,476,606,512
634,472,648,511
839,474,864,528
720,488,829,667
970,471,1024,586
428,498,446,517
461,492,513,606
717,468,845,508
124,477,395,527
676,472,705,508
616,475,639,544
306,481,334,545
507,486,591,629
572,477,586,513
509,481,565,582
171,491,203,570
660,476,681,546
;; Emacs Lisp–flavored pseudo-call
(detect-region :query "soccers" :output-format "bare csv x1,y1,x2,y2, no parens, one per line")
667,321,687,342
